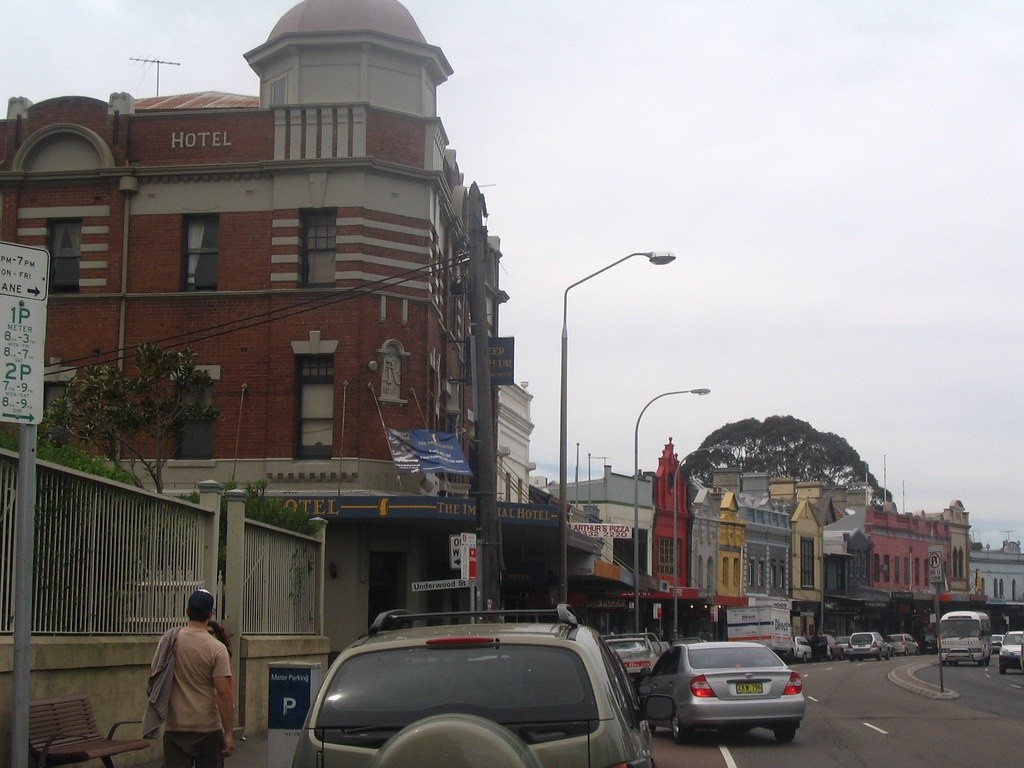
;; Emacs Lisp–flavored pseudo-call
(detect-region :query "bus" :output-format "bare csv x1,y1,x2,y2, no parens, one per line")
933,611,991,668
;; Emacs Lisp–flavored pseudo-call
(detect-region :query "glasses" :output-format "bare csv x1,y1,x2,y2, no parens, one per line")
207,630,215,635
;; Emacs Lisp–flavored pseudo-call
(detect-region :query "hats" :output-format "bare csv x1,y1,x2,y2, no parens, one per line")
188,589,214,612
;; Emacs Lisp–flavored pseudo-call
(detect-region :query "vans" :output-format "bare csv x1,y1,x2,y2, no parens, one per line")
996,631,1024,675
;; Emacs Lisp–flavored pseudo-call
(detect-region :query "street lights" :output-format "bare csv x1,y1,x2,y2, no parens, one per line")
560,249,679,604
672,444,734,641
819,481,869,635
633,388,712,633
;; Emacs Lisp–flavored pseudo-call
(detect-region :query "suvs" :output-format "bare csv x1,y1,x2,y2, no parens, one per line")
844,632,890,661
809,635,851,664
600,632,708,689
290,605,676,767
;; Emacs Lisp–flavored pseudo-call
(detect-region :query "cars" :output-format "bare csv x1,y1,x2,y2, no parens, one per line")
990,633,1005,653
919,634,938,655
885,633,920,657
794,635,812,663
636,640,806,745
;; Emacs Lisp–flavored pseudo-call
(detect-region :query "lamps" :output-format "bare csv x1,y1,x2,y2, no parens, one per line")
328,562,337,579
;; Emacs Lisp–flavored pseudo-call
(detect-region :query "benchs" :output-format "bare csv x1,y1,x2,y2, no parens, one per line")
29,693,152,768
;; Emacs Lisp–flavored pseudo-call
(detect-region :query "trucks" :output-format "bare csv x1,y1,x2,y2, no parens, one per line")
726,606,796,664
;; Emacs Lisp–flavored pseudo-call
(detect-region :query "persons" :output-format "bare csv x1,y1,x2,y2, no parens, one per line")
609,622,665,642
802,631,828,662
157,590,235,768
